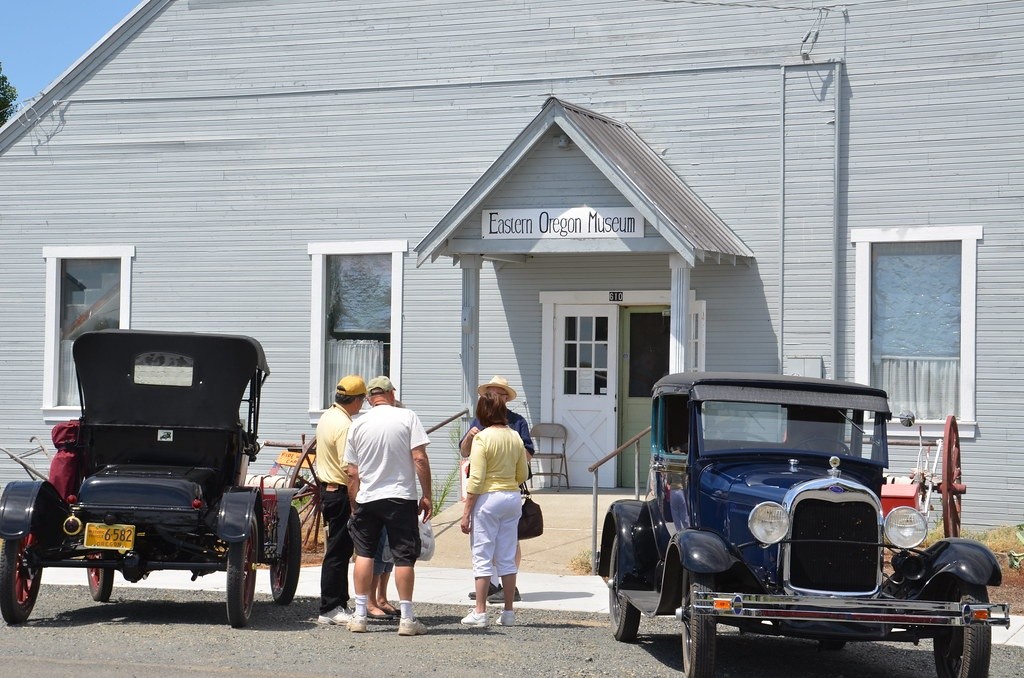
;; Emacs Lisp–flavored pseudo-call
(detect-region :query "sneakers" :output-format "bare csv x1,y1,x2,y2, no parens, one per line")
345,614,369,632
461,607,488,628
318,605,354,627
494,609,516,627
399,618,428,635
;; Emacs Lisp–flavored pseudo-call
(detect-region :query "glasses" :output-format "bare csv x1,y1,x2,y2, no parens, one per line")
496,392,509,397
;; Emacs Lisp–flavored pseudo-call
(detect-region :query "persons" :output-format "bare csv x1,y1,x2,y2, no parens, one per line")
315,375,433,636
459,376,535,628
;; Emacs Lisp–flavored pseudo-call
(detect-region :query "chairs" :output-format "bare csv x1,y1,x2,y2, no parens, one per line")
529,423,569,492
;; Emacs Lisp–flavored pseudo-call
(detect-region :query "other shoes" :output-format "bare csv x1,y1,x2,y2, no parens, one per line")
377,603,401,615
366,602,393,620
468,581,503,599
347,603,355,615
487,587,521,602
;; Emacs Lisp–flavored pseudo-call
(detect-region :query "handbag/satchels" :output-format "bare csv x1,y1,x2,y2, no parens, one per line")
518,495,543,540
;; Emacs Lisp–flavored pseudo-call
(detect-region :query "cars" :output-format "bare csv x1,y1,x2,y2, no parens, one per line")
594,370,1011,678
0,329,302,627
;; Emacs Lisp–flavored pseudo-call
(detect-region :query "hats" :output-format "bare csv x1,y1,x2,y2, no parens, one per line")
336,375,367,395
477,375,517,400
367,376,397,396
